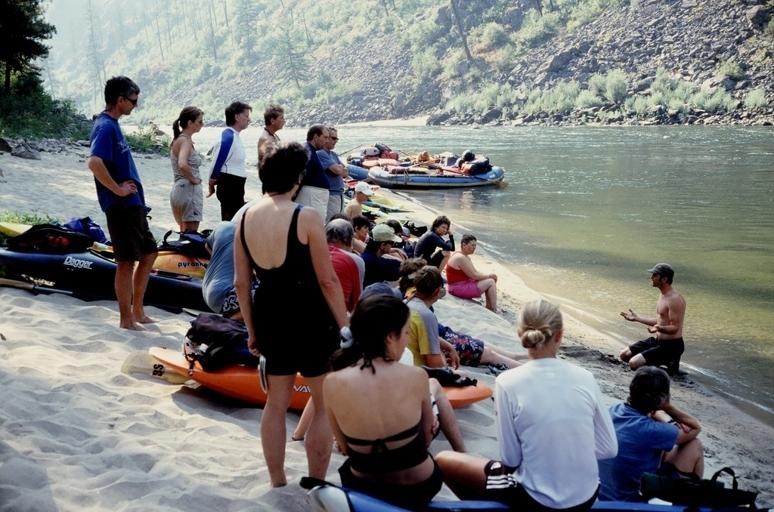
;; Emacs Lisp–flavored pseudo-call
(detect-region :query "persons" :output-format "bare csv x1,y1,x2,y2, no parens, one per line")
619,262,686,371
203,220,260,321
257,105,286,175
319,292,443,507
593,367,705,503
435,297,618,508
326,179,528,387
167,103,204,236
205,101,253,224
87,75,158,332
296,125,329,234
232,140,351,491
317,128,348,217
416,151,434,164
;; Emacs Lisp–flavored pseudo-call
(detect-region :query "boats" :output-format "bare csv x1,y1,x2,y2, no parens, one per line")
345,137,509,193
292,473,740,511
0,213,221,310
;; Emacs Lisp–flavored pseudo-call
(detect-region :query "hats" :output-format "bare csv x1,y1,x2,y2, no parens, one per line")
354,181,376,198
369,223,403,243
323,219,356,246
645,262,676,281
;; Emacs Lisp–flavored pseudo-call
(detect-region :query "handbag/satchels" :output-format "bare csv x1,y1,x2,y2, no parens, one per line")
680,468,758,512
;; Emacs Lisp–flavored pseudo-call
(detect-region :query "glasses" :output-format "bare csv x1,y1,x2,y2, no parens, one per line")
331,136,338,140
125,97,137,105
